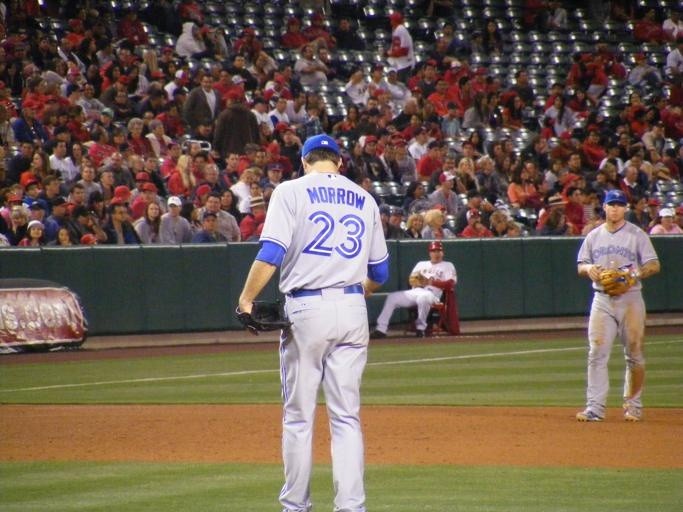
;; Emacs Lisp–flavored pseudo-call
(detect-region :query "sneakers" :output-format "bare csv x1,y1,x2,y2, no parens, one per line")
416,329,425,338
624,407,641,421
370,331,386,339
576,410,603,421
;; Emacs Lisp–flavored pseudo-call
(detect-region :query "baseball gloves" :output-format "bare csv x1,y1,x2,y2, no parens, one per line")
409,271,424,288
600,269,636,296
235,300,293,332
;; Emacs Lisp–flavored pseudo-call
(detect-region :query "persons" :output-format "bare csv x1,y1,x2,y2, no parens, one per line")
0,1,682,253
575,189,661,423
366,241,458,340
237,133,392,511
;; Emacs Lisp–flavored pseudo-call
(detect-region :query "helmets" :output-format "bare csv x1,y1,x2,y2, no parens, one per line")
429,242,443,251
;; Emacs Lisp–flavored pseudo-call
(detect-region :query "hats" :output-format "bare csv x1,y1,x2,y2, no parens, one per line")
301,135,340,156
222,74,294,207
565,174,582,184
467,209,483,222
379,204,390,215
22,69,189,118
8,172,216,245
289,13,325,25
605,190,627,205
388,13,401,24
434,204,446,210
647,199,683,216
336,60,462,148
439,171,455,182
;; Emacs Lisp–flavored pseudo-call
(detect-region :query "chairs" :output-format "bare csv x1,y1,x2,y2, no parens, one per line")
404,280,455,338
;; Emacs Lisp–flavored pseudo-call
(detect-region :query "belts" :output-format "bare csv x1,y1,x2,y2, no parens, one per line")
286,284,363,298
